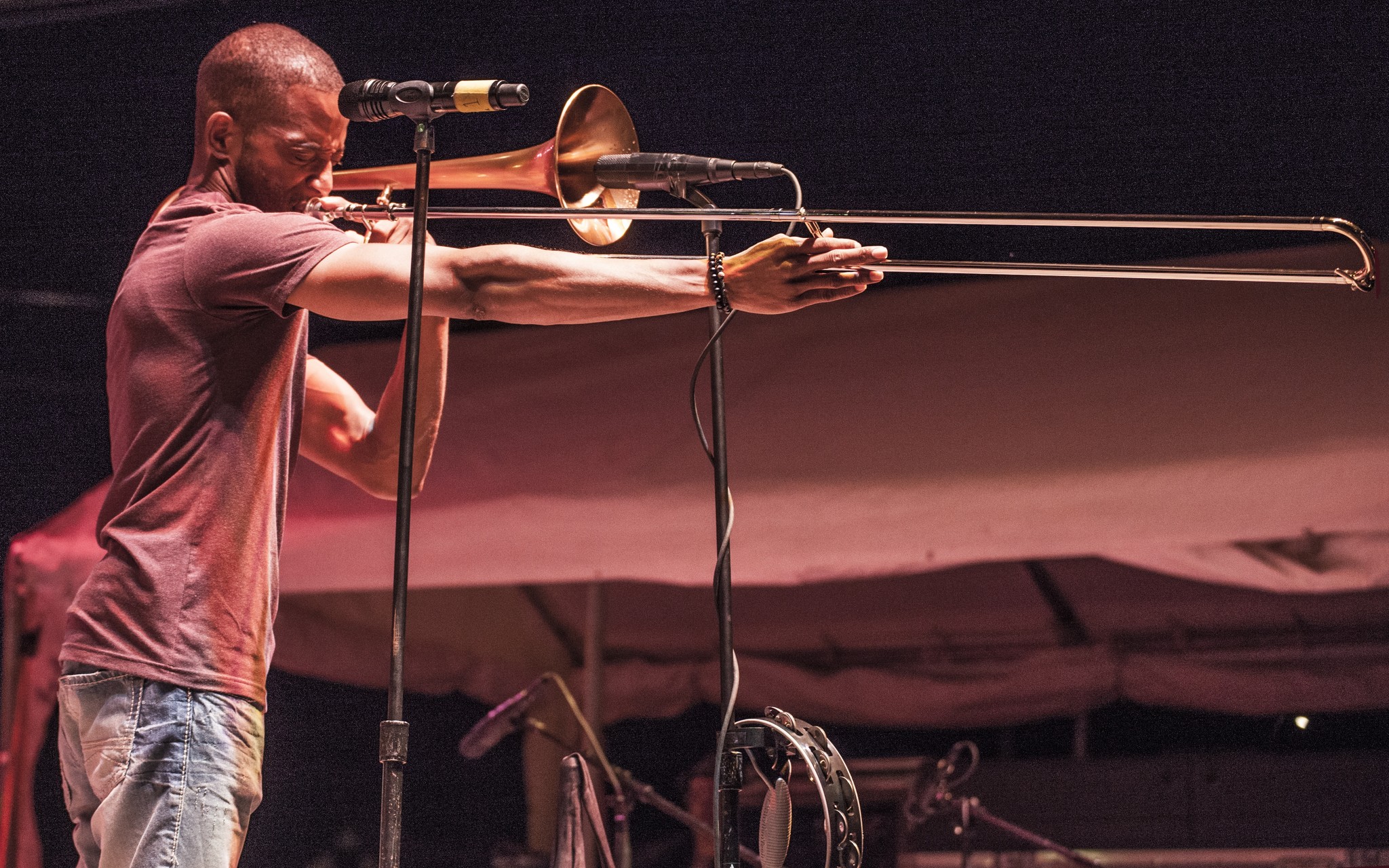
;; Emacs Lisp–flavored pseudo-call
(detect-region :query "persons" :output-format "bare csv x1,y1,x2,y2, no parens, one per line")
50,17,891,868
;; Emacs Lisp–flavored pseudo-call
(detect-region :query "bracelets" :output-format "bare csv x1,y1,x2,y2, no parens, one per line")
715,251,732,315
708,252,724,314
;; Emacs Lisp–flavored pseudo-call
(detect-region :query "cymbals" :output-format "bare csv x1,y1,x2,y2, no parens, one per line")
731,703,868,868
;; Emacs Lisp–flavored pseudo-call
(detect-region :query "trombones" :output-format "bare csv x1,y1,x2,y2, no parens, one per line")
298,80,1382,298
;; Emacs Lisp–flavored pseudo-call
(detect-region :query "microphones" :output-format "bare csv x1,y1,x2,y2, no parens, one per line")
906,756,939,824
758,778,792,868
337,78,530,124
459,680,541,759
